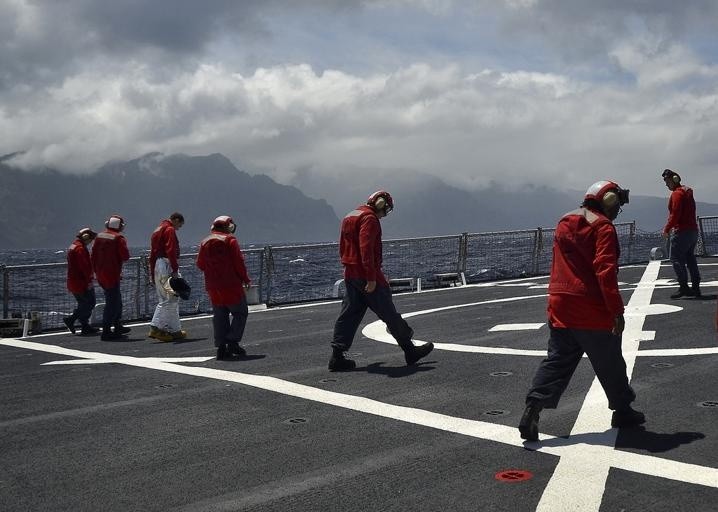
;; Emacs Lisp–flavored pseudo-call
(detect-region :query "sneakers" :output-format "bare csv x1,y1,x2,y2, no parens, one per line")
147,328,186,341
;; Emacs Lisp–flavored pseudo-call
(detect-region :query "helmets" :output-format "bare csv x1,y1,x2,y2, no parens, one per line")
662,168,681,184
366,190,395,217
104,214,126,232
584,181,630,210
76,227,98,242
210,216,236,233
162,277,191,299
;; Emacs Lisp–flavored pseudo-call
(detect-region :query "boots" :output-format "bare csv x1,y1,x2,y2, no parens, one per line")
400,341,435,364
519,397,546,440
611,406,645,427
63,309,131,340
670,279,702,299
328,346,356,370
216,341,245,358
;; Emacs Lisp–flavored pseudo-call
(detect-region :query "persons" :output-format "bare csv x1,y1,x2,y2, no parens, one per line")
148,212,188,341
518,181,647,439
328,191,433,370
92,215,130,342
662,169,701,298
63,228,98,336
196,216,251,361
